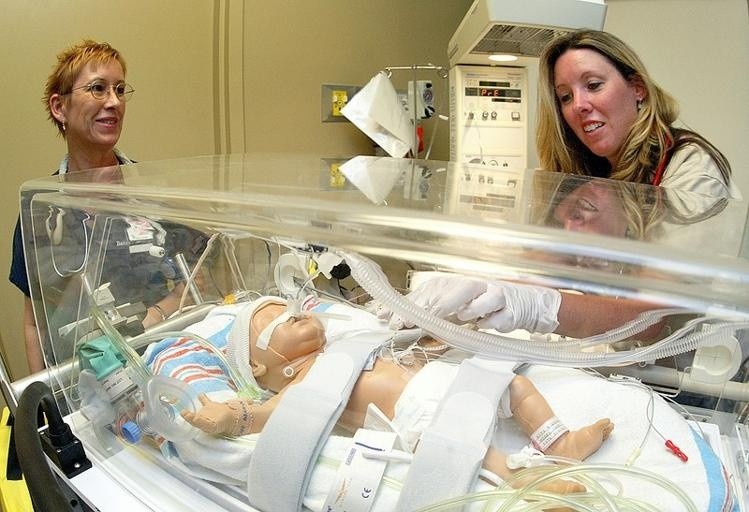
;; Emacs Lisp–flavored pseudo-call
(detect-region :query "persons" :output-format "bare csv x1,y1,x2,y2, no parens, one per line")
524,169,668,280
375,28,748,411
179,295,615,512
9,38,220,377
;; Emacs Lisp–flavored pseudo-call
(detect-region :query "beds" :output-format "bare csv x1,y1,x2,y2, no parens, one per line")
0,289,749,511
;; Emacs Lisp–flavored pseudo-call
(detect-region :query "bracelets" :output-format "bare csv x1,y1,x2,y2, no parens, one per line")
150,304,168,321
224,400,254,437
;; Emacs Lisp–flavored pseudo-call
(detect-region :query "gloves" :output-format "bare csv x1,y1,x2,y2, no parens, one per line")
378,274,486,327
460,276,562,339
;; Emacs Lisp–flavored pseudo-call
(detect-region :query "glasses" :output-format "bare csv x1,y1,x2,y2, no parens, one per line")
55,78,135,103
